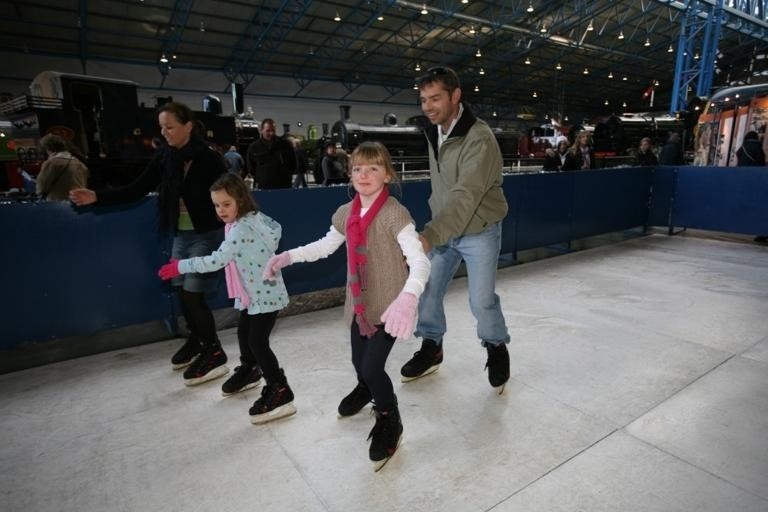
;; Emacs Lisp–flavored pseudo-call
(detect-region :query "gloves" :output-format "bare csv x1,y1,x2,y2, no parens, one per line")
265,251,291,278
158,258,181,281
381,292,419,340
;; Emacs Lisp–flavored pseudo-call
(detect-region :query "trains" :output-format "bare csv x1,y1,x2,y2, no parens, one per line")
0,67,693,193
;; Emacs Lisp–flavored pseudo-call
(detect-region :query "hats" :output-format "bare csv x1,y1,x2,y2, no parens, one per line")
556,135,571,147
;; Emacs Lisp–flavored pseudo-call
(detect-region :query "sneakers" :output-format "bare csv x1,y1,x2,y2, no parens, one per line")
249,368,294,415
483,341,510,387
171,324,227,379
366,394,403,461
222,357,262,393
401,338,443,378
338,377,372,416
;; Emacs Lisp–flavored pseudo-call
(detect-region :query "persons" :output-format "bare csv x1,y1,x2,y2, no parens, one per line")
69,102,230,380
570,130,593,170
157,172,294,415
35,134,89,202
264,141,432,461
736,131,765,166
658,133,684,166
542,136,573,171
223,119,345,190
400,67,510,388
636,137,658,165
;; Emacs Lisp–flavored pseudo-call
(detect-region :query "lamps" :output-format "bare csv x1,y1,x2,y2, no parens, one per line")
160,53,168,63
333,10,341,22
